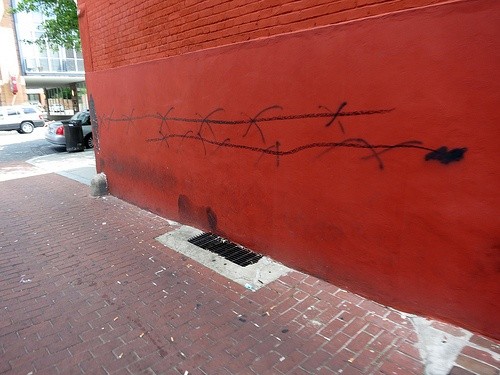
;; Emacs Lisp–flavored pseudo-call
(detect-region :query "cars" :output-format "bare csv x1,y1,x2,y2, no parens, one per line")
44,111,94,149
48,104,64,112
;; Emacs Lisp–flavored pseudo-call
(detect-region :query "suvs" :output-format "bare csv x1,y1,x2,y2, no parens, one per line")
0,105,45,134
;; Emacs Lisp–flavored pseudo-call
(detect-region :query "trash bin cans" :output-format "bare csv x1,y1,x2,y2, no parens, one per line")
61,119,85,153
